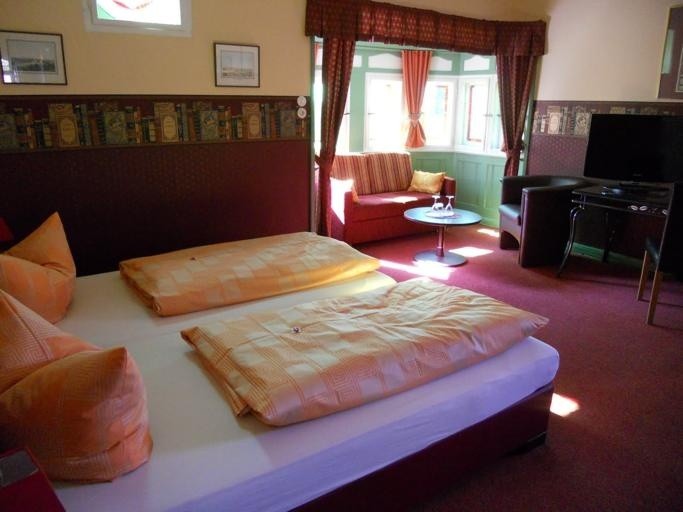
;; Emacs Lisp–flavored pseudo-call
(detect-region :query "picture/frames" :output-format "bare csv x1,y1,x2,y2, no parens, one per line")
214,42,260,88
0,29,68,85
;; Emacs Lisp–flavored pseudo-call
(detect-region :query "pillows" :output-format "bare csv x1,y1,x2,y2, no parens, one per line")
407,170,446,195
330,176,360,204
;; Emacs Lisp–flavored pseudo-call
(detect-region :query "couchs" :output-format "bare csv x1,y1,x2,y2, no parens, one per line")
315,152,455,247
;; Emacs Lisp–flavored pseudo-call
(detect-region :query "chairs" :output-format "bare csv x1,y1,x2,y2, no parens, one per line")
499,175,580,270
635,181,683,325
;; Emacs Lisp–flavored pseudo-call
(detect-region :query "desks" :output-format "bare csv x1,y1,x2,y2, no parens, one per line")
557,183,673,280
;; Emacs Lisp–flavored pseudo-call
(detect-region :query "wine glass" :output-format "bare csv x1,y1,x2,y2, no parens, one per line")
430,194,457,214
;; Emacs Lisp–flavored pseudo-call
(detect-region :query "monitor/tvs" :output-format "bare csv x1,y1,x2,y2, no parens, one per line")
584,114,683,195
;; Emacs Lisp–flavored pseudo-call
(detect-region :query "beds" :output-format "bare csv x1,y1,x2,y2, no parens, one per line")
0,231,560,511
